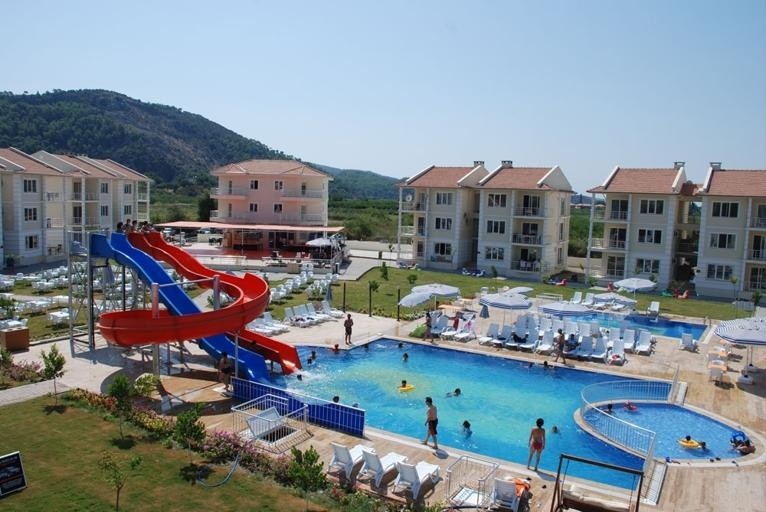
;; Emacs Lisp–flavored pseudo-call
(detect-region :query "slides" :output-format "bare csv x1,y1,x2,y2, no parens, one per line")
91,232,302,386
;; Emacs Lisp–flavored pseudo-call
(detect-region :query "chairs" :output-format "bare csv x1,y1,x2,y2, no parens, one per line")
391,458,441,498
358,448,407,490
324,438,377,477
492,472,532,512
398,274,741,392
0,228,347,350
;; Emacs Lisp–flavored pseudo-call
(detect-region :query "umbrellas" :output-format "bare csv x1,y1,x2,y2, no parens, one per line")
714,316,766,377
398,277,657,332
305,236,333,260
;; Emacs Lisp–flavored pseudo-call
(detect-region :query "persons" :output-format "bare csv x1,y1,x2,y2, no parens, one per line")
248,340,257,352
421,396,439,449
217,352,231,391
726,438,755,455
525,418,546,472
394,302,445,364
400,382,408,389
624,401,634,410
676,435,707,449
293,311,371,408
462,418,471,439
115,217,156,233
603,403,614,415
524,329,579,372
447,387,463,400
